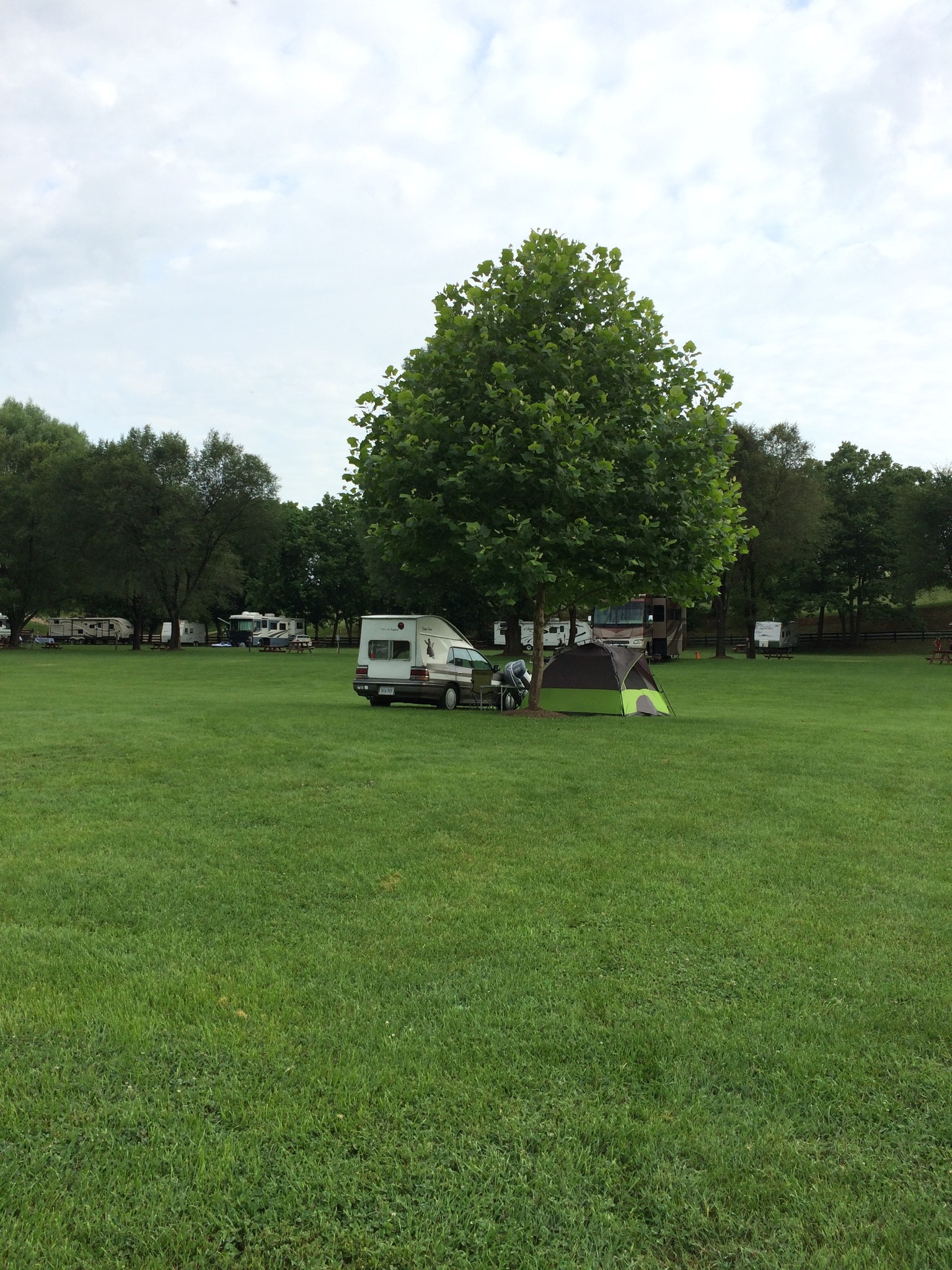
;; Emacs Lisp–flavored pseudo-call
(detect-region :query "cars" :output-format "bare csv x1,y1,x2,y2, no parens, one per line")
291,634,312,648
352,614,519,711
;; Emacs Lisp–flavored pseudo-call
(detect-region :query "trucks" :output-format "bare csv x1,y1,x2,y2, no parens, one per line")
47,616,134,645
161,620,206,647
0,612,11,641
587,593,688,663
229,611,306,648
494,617,592,651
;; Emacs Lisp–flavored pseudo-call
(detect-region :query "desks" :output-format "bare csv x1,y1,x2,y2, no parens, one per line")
288,641,312,654
734,644,747,653
0,640,8,650
479,685,524,713
260,645,284,651
768,650,790,660
152,643,170,651
44,643,61,650
930,650,952,665
34,638,55,645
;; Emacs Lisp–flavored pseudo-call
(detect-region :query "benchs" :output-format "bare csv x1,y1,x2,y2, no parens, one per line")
286,647,316,651
924,657,952,661
41,647,63,649
0,644,7,646
732,647,736,651
259,650,286,652
148,647,165,649
764,655,794,659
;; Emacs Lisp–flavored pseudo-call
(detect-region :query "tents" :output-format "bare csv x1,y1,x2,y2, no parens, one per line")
518,637,677,717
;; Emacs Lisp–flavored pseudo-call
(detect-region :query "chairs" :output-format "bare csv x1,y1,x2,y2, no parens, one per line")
469,669,501,711
